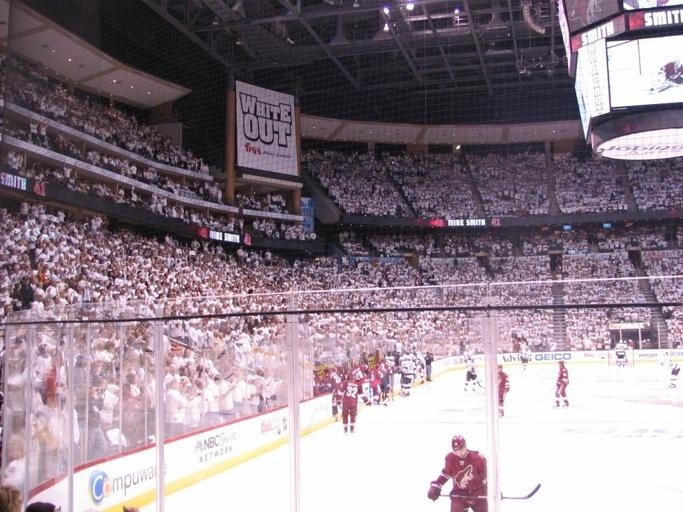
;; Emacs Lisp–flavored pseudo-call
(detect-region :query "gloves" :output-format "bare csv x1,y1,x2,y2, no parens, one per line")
428,481,442,501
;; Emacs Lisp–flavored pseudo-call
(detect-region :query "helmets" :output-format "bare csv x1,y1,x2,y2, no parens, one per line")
452,436,465,451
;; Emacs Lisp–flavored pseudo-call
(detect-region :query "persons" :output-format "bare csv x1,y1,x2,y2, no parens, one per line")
2,1,683,512
